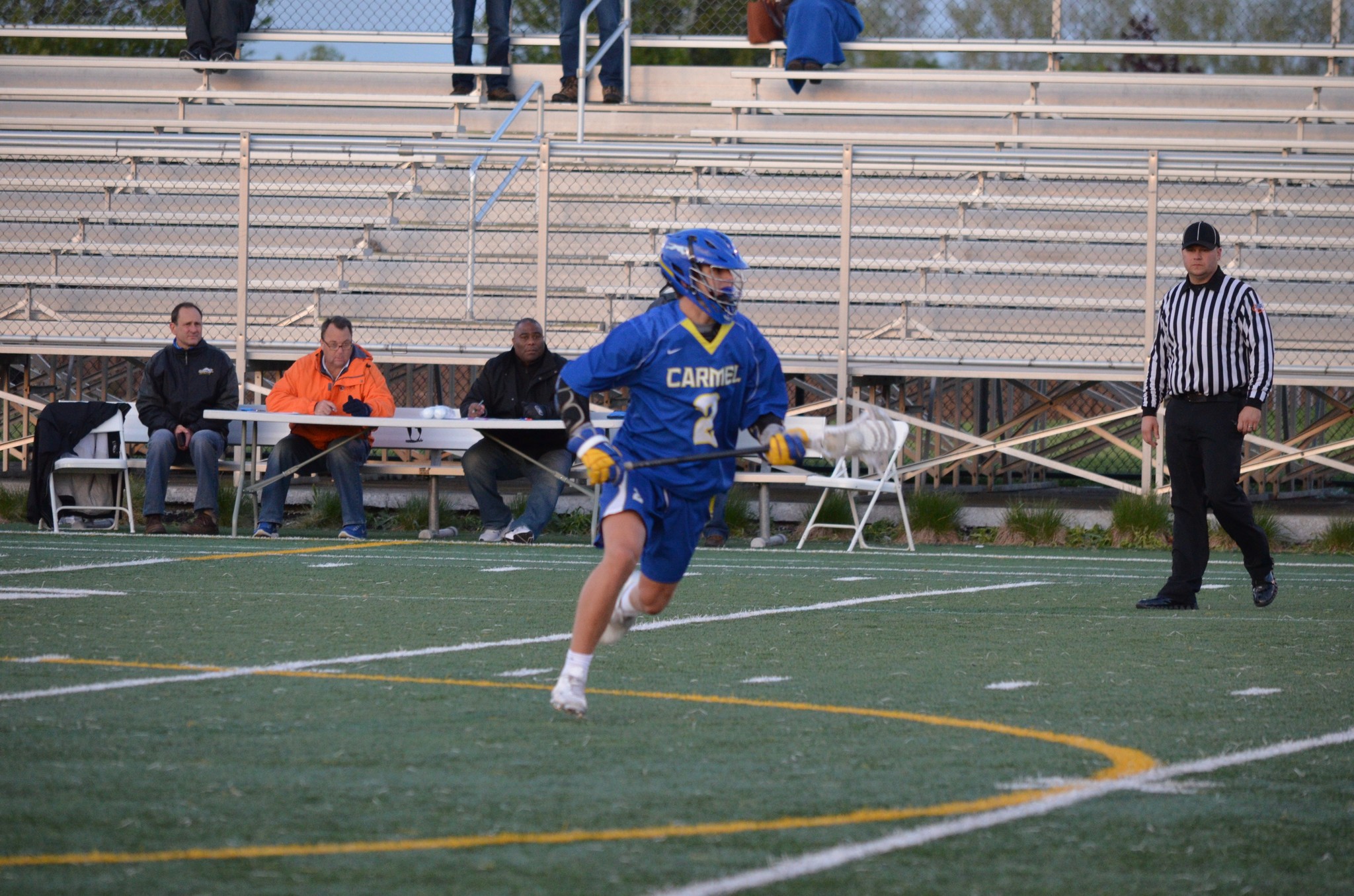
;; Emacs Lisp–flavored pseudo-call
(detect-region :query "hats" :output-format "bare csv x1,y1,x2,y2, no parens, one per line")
1182,221,1220,250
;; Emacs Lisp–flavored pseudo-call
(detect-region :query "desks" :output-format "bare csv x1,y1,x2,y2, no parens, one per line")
203,410,625,546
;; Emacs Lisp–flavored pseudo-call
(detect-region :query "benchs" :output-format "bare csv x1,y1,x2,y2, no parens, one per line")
58,400,826,548
0,26,1354,371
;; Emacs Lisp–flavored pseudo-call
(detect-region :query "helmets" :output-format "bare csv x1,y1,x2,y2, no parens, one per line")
659,228,750,325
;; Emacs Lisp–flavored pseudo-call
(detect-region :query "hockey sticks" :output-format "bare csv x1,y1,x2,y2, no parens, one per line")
570,407,896,480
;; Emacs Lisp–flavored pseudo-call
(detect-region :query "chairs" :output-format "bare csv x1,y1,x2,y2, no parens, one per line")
26,401,137,534
797,421,916,552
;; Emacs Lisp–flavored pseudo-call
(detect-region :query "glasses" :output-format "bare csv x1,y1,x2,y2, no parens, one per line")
322,337,352,351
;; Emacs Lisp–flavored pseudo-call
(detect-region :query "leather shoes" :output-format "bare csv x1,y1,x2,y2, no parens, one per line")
1136,596,1199,609
1252,556,1278,606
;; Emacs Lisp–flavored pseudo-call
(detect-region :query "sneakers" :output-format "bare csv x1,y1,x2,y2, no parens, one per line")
600,573,640,644
180,512,219,535
479,518,514,542
254,522,279,537
705,535,724,547
338,523,367,539
145,514,166,534
550,675,588,719
504,526,534,543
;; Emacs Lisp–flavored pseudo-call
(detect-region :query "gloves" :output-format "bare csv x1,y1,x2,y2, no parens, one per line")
343,395,372,417
521,402,546,420
760,423,809,466
567,427,625,487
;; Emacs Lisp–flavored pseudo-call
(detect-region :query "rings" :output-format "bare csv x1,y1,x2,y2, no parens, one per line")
1248,426,1253,428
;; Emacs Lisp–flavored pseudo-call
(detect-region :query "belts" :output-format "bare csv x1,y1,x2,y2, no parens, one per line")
1173,392,1244,402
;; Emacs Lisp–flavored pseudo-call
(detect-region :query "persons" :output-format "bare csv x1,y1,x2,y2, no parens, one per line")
450,0,516,103
1136,221,1277,610
776,0,863,87
552,0,624,103
460,318,589,544
252,316,395,539
551,229,808,715
135,302,239,534
180,0,258,75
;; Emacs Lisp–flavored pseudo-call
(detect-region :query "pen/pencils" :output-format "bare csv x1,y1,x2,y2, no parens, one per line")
240,408,259,411
466,417,487,420
331,408,334,411
473,400,484,413
